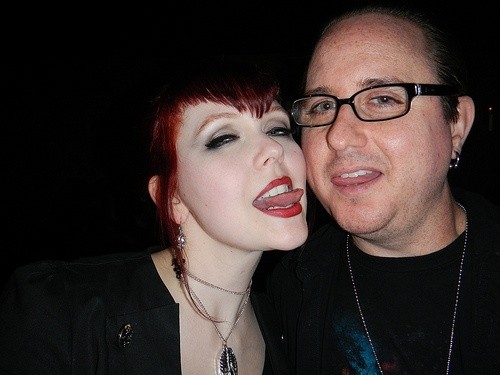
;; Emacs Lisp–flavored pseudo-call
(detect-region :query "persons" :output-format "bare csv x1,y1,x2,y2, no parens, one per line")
261,7,500,375
1,52,309,374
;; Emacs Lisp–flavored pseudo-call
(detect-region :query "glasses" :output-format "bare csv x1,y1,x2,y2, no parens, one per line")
290,83,457,127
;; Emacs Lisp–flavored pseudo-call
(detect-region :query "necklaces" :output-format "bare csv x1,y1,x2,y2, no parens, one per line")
346,198,469,375
170,248,255,375
